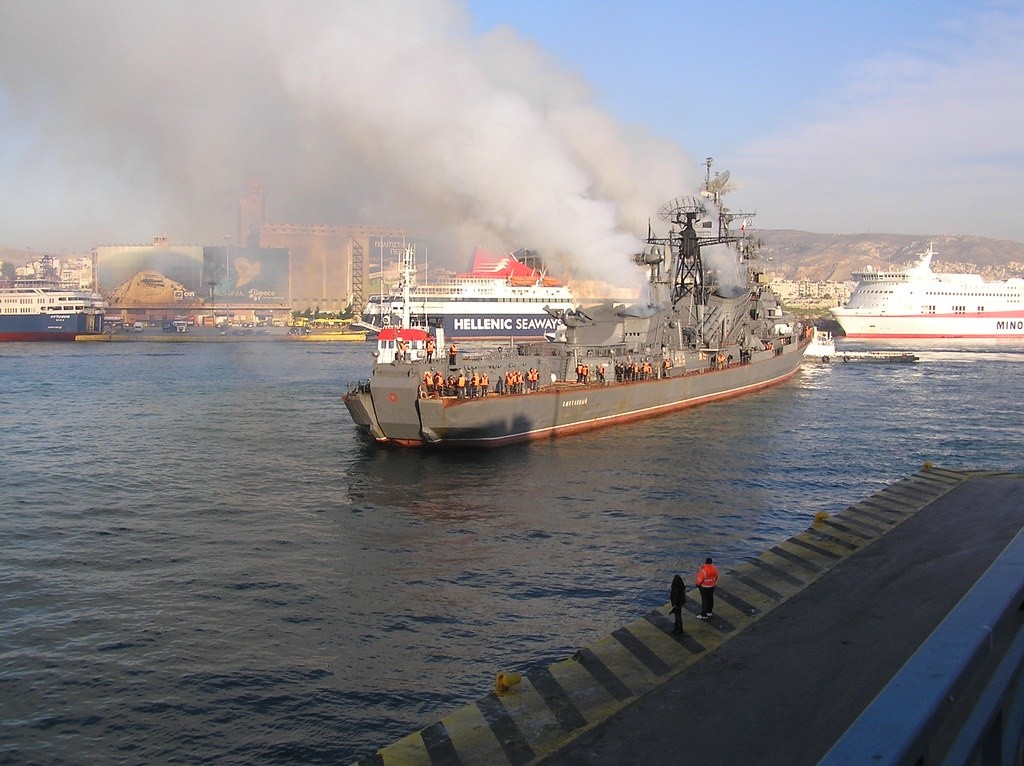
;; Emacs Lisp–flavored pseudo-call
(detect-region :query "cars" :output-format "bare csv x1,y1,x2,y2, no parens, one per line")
105,317,313,337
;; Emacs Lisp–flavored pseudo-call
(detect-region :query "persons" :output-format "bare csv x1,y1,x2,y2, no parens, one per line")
696,558,719,619
399,339,733,398
669,575,687,635
766,342,774,351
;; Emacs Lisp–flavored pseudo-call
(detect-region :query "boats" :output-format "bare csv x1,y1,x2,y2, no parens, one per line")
350,244,575,340
803,331,837,357
0,280,109,342
828,238,1024,341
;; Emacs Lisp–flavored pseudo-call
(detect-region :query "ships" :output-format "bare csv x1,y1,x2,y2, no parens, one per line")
336,153,816,450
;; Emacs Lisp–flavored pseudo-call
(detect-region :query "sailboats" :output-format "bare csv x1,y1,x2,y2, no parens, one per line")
374,242,439,363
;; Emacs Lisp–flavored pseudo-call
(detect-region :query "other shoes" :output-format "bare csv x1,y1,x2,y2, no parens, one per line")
706,612,713,616
696,614,709,619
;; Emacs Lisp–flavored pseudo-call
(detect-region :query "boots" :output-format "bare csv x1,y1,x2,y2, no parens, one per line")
673,623,682,633
